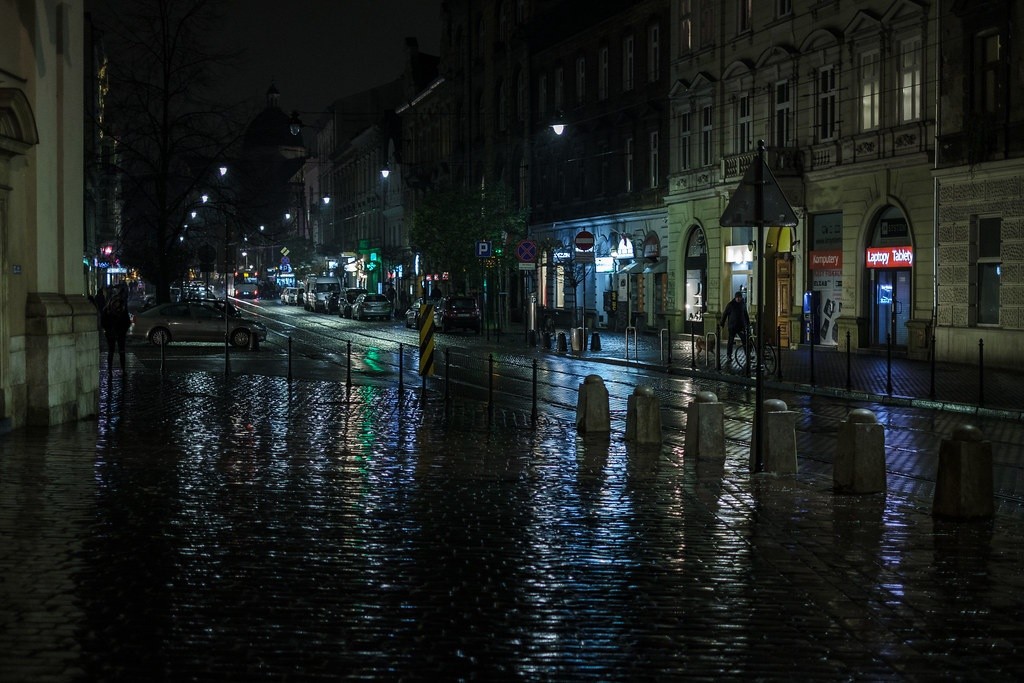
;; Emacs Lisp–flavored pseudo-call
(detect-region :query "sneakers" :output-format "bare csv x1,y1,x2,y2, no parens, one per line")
727,354,733,361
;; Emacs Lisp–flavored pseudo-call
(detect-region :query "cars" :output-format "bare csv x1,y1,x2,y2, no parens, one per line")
404,298,440,330
281,286,305,306
145,280,239,318
324,290,341,315
125,302,268,350
433,294,482,337
351,292,392,322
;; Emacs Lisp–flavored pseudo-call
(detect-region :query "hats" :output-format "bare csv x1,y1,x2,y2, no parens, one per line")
735,292,742,298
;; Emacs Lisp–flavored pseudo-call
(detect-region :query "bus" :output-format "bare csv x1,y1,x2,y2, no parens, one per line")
223,269,259,299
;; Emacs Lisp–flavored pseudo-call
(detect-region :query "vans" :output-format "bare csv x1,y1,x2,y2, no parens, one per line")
338,287,369,319
303,276,342,313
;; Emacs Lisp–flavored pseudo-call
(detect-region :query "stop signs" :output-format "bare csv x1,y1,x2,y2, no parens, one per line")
575,230,594,250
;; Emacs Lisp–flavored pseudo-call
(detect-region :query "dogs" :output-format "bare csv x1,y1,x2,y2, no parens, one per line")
696,337,716,358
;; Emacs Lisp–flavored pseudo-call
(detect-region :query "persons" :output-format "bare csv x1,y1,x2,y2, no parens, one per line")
430,284,442,299
719,291,755,363
87,277,138,377
262,280,294,299
388,285,397,312
399,290,408,310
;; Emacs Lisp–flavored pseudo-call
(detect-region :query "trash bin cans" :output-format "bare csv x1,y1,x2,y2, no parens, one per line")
570,326,588,351
